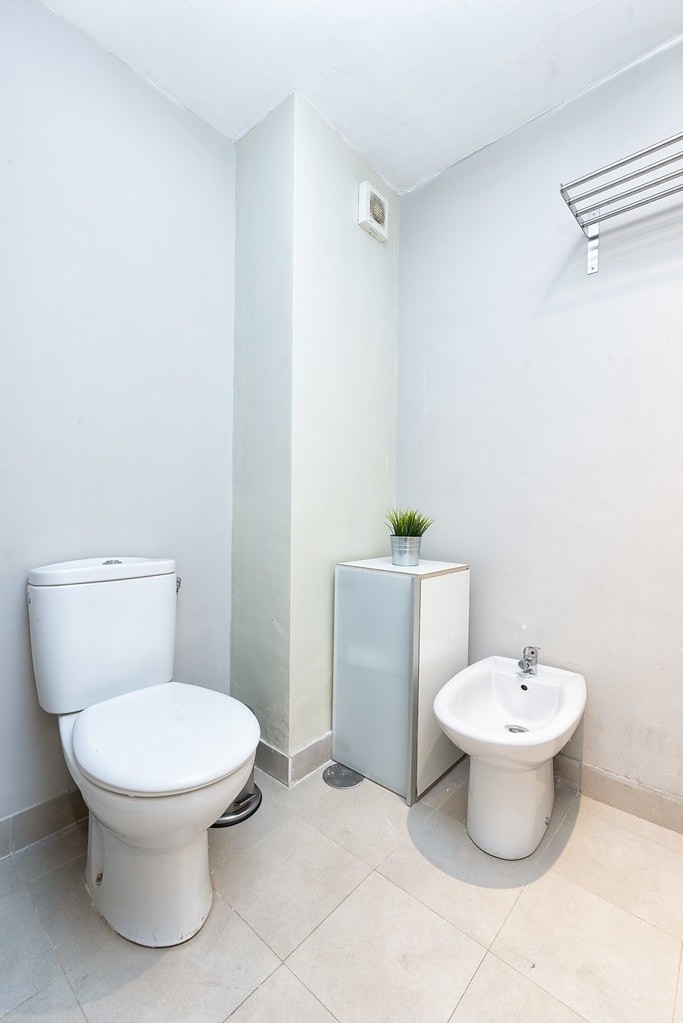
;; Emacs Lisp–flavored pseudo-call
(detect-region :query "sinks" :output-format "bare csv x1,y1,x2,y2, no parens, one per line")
433,656,588,861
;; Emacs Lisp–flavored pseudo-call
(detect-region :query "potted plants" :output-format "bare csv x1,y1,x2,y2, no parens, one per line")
382,505,436,567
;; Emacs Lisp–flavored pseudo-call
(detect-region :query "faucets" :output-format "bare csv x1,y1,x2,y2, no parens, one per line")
517,646,541,675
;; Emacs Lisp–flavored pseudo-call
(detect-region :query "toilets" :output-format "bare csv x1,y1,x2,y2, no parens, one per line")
27,558,261,948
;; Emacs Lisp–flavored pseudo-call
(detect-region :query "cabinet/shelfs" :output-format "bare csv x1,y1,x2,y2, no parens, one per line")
331,557,471,807
